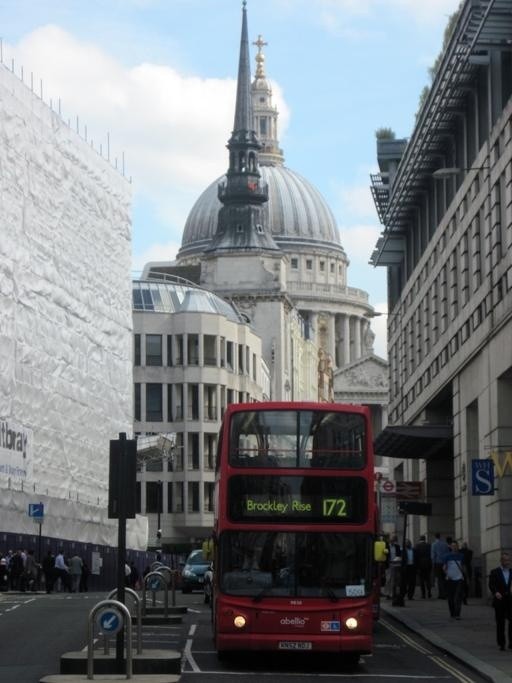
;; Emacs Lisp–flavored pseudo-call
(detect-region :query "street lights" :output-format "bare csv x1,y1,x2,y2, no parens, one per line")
156,478,163,558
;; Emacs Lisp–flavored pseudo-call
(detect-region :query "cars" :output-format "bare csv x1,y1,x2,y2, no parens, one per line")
202,559,213,605
177,547,215,593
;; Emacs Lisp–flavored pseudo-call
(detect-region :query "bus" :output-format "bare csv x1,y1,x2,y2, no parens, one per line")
371,503,383,620
203,402,388,660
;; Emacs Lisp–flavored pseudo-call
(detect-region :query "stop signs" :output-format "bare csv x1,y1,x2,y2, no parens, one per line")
382,481,394,493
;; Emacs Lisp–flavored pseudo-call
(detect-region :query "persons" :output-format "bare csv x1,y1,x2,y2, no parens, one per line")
375,531,482,620
0,548,92,594
124,562,149,589
488,553,512,652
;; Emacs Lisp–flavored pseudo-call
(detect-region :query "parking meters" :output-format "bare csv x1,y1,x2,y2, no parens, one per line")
390,555,409,605
